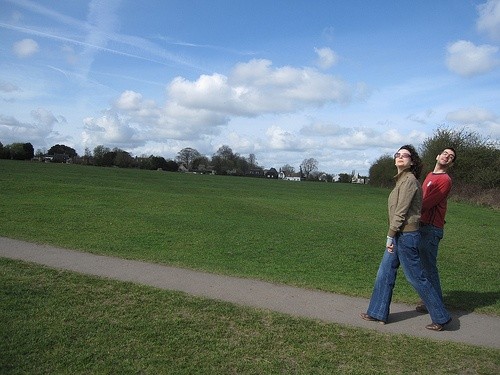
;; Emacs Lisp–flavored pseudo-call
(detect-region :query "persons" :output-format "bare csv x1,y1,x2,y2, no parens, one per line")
415,147,457,314
360,144,453,331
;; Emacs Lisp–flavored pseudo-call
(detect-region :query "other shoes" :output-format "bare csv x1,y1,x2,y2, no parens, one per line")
416,305,428,313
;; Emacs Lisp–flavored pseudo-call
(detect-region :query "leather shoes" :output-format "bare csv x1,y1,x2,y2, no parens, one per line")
424,316,452,332
360,312,385,325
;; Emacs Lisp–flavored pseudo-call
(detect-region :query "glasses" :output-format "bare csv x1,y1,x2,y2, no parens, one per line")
394,153,412,158
